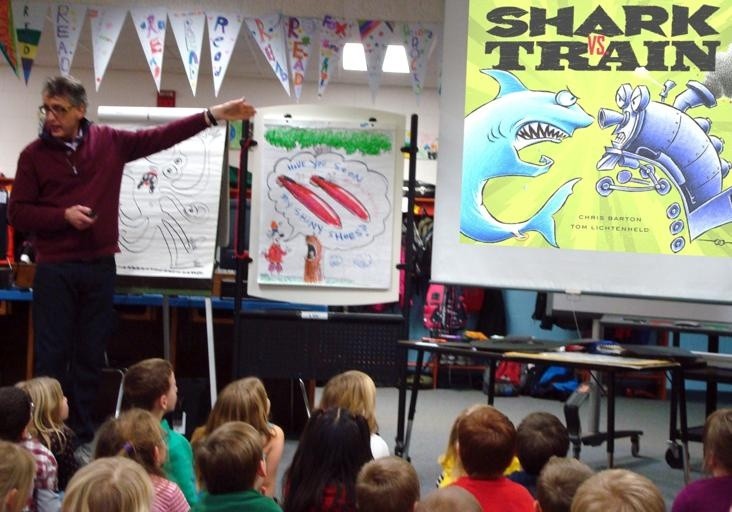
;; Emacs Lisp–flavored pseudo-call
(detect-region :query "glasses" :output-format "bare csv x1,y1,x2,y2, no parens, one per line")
38,105,76,117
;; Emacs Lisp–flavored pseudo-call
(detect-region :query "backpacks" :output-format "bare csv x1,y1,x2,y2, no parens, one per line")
416,208,434,281
494,359,580,400
422,285,487,330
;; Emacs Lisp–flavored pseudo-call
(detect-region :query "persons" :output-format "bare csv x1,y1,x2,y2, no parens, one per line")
281,371,666,512
7,76,258,440
672,409,731,512
1,358,285,512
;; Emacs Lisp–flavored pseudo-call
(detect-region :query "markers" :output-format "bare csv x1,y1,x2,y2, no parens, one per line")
422,336,447,343
439,334,469,341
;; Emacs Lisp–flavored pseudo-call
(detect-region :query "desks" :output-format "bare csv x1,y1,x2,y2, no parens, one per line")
397,336,691,473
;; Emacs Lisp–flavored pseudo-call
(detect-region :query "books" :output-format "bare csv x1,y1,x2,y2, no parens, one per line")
595,341,696,361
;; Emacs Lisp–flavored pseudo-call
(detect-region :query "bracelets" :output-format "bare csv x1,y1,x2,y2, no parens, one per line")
206,108,218,126
